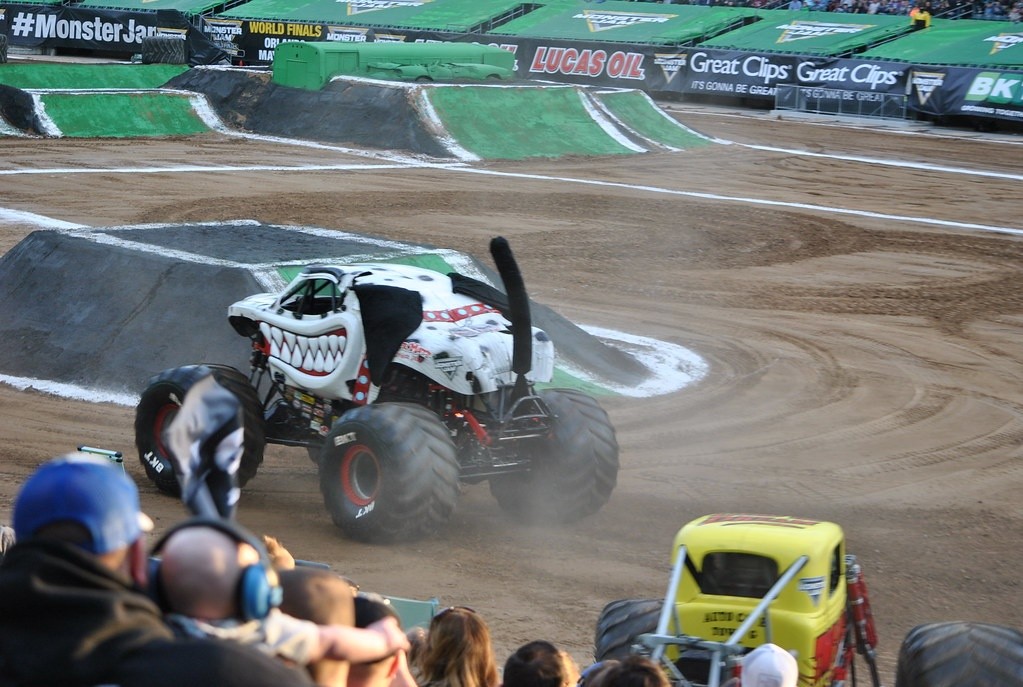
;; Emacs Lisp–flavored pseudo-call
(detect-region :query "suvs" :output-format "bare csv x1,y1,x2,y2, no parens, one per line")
133,235,622,544
595,513,884,686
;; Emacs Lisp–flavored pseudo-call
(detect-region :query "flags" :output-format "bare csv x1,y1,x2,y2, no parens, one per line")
163,372,243,522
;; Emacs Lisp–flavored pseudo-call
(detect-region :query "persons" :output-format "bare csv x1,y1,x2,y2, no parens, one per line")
159,527,408,665
910,7,930,32
706,0,1023,23
740,643,798,687
0,452,669,687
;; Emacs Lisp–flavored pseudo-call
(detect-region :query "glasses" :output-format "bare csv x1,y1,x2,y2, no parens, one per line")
430,605,475,627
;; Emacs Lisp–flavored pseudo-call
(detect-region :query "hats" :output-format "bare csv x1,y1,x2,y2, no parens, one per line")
735,643,798,687
13,450,155,554
